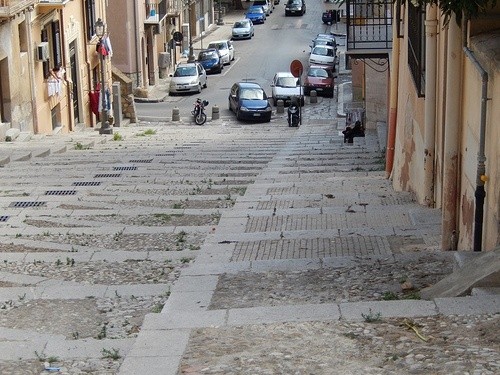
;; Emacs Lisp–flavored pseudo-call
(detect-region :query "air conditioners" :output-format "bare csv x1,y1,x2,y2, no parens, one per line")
37,42,49,60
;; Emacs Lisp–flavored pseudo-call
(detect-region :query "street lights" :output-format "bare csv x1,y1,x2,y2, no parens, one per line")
184,2,196,63
216,0,225,25
93,17,114,135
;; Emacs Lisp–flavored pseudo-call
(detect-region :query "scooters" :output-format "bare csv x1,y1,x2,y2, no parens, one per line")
285,100,301,127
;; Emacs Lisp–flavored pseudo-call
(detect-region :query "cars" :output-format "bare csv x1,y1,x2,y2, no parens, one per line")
231,18,255,40
253,0,273,17
169,62,209,96
194,48,224,75
309,37,340,53
333,9,337,24
315,33,336,42
322,9,339,25
270,71,305,107
283,0,307,17
207,40,235,66
308,44,337,73
302,64,338,99
228,80,273,123
243,5,267,25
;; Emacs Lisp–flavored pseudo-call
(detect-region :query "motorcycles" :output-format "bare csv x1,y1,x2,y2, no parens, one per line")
189,97,210,126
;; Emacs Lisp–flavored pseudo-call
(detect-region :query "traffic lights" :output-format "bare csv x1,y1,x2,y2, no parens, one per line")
326,10,333,26
